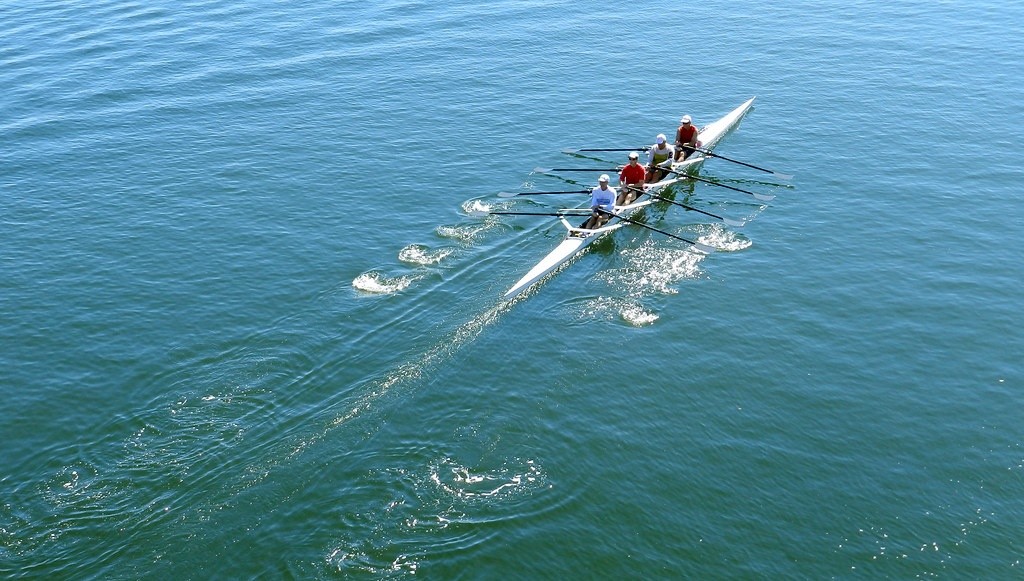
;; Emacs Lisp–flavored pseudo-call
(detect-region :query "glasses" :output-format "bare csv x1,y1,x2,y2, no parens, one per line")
683,123,688,124
629,158,635,160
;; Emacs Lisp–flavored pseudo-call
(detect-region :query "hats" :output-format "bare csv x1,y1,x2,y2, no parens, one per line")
629,152,639,160
598,174,610,182
681,115,692,124
657,134,666,144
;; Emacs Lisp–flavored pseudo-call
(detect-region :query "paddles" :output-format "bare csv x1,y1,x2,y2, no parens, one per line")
497,190,592,199
561,146,650,154
625,186,745,227
678,143,793,181
469,210,594,218
587,186,626,190
533,166,622,174
653,165,775,202
596,207,717,253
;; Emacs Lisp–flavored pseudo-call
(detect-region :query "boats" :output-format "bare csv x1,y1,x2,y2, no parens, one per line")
503,96,757,300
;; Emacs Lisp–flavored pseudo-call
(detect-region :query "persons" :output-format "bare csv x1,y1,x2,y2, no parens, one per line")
616,152,645,206
587,174,617,229
674,115,698,163
645,134,675,183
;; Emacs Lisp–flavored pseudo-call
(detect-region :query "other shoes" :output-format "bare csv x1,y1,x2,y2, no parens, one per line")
576,233,590,237
